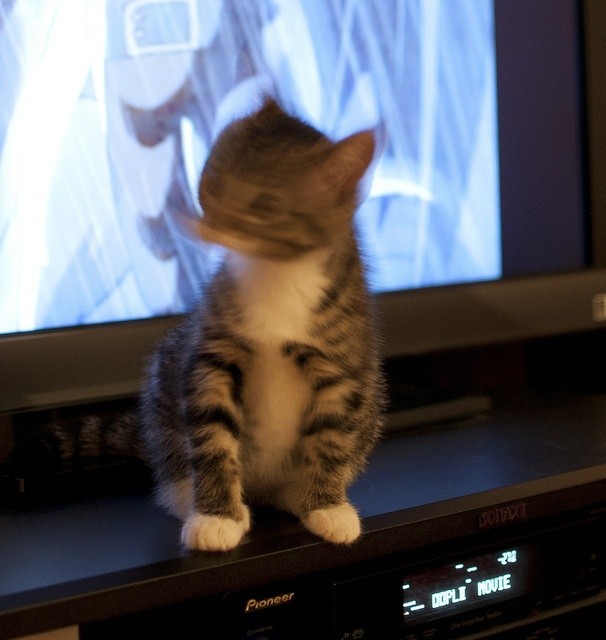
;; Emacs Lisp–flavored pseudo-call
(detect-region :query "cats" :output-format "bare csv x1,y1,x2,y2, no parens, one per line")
138,91,390,554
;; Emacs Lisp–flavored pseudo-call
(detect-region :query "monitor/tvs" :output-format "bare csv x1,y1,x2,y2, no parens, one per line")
0,0,604,442
